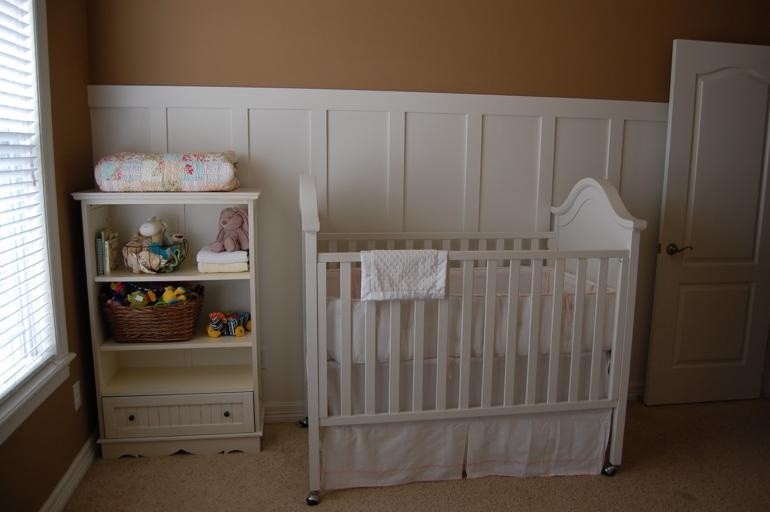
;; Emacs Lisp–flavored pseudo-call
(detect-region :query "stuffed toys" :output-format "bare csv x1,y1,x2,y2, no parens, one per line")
208,206,249,254
111,283,200,306
204,311,252,337
139,215,170,260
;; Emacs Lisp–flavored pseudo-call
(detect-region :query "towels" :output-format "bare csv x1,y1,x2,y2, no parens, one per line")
358,242,451,304
194,246,249,275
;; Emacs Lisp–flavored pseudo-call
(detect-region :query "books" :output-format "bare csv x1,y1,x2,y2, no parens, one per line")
96,229,122,274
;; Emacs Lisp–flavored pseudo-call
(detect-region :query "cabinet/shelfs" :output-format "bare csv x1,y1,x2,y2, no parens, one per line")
71,182,270,459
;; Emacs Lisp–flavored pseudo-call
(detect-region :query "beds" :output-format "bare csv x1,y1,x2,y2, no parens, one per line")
291,167,652,505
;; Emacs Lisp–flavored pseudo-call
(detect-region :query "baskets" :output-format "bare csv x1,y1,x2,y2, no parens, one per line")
103,293,204,344
